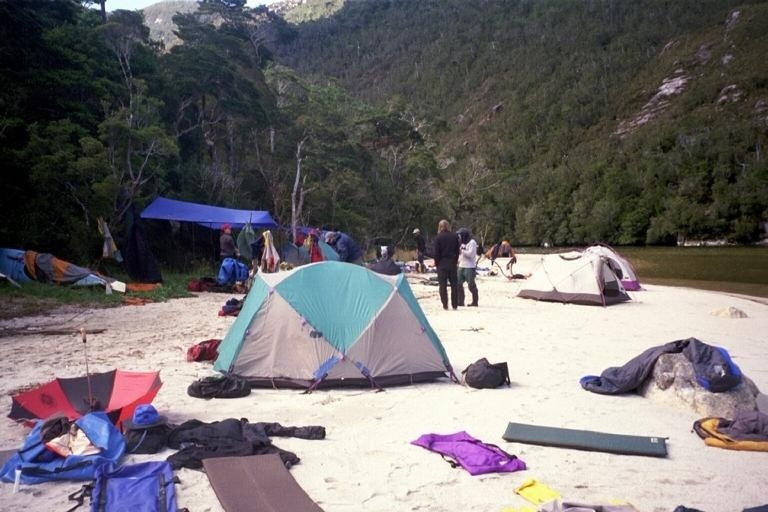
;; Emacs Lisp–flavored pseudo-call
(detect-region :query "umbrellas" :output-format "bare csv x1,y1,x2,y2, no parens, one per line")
7,327,164,432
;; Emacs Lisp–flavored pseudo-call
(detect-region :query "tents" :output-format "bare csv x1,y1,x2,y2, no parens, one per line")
216,260,460,394
516,251,630,308
585,243,641,291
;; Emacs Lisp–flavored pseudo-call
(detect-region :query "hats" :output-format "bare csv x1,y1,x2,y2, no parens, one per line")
124,405,166,431
221,224,231,230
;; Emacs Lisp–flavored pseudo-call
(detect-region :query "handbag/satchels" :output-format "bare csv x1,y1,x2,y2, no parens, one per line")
461,358,510,388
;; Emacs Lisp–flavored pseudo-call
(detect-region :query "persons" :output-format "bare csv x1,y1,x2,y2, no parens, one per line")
220,224,238,263
456,227,479,306
414,229,426,262
485,244,500,266
499,238,516,262
435,219,458,310
326,231,362,265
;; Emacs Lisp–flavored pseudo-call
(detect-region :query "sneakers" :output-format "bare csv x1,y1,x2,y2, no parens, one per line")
443,303,478,309
